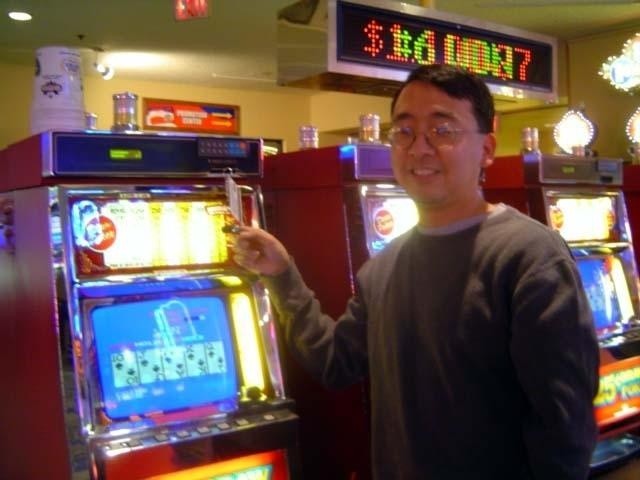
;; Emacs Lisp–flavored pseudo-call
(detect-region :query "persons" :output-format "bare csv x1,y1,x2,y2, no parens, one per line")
231,62,601,479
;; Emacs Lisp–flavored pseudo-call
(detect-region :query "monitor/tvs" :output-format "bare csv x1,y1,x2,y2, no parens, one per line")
566,242,640,349
81,286,244,434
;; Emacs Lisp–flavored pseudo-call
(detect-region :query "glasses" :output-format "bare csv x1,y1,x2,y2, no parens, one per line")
389,123,474,148
